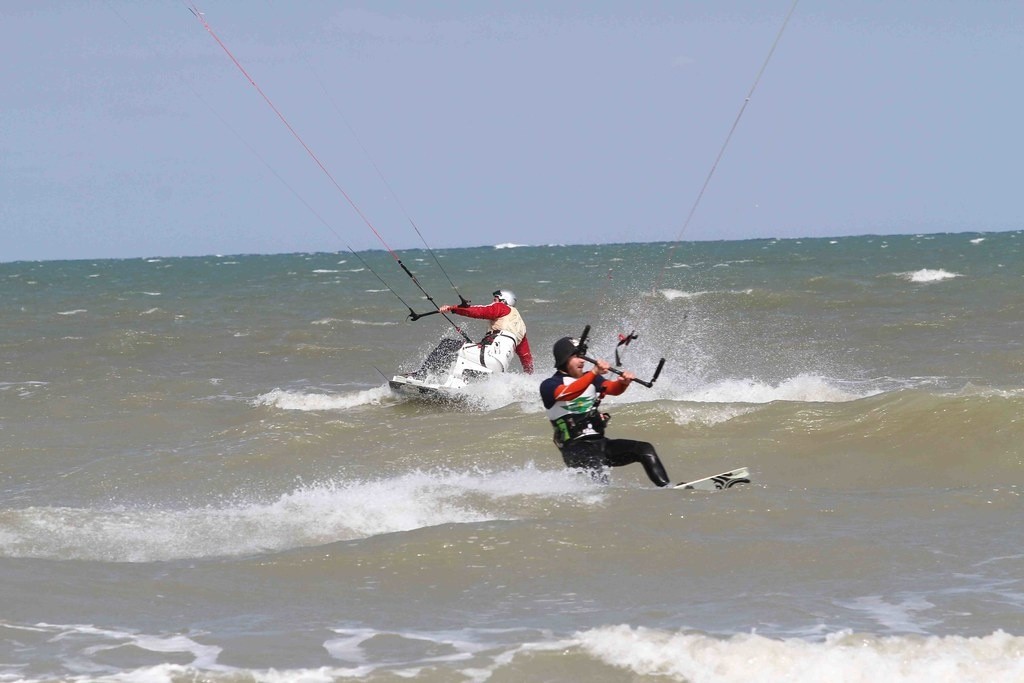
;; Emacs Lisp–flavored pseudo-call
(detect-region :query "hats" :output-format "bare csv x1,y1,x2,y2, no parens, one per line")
553,337,588,369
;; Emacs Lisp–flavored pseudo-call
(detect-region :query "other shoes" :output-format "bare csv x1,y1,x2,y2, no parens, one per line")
398,371,428,383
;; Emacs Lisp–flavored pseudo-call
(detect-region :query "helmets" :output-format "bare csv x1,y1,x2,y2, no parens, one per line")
493,289,517,307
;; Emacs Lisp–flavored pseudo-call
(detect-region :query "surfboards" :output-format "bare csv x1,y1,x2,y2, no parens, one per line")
387,375,473,405
670,466,752,492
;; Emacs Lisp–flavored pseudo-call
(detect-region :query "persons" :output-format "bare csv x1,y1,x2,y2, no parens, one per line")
539,337,685,489
401,290,534,385
